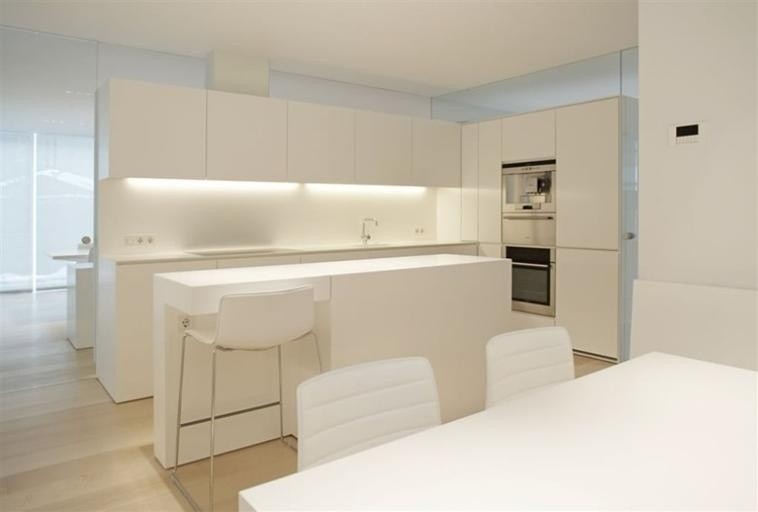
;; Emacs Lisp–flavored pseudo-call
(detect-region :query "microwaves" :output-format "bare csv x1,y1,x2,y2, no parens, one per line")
501,158,557,215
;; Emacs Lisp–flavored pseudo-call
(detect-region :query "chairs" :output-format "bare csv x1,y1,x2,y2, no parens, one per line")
174,288,321,512
486,326,574,409
295,357,440,472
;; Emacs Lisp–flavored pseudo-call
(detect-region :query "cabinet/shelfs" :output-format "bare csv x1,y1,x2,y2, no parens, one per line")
287,100,355,183
413,117,461,186
93,259,217,403
502,108,556,164
355,110,413,186
217,255,301,269
462,119,502,243
556,96,637,363
206,91,287,182
96,77,206,181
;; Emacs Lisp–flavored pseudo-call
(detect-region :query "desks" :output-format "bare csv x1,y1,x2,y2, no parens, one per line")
238,352,758,512
67,262,94,350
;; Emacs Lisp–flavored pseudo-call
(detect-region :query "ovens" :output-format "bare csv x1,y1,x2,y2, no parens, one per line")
501,243,557,318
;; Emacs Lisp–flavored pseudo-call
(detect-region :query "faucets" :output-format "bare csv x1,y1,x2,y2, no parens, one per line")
360,217,378,243
